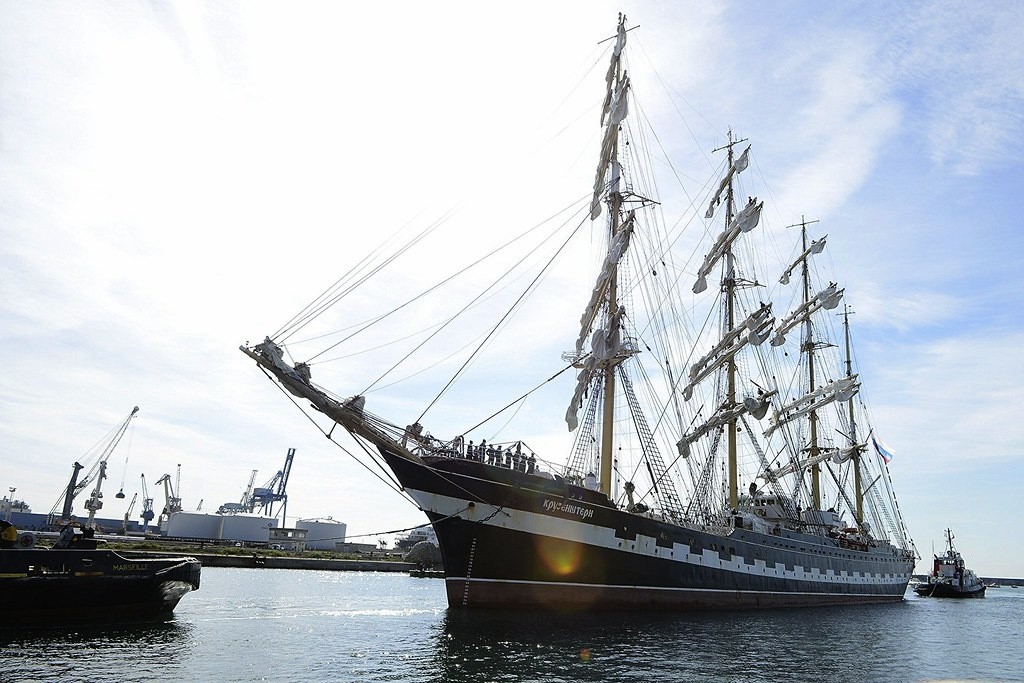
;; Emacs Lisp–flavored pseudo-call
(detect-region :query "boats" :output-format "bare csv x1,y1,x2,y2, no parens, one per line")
911,527,988,599
0,515,203,630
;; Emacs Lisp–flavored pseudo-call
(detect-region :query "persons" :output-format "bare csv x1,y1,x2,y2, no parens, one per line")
0,518,18,549
467,438,536,476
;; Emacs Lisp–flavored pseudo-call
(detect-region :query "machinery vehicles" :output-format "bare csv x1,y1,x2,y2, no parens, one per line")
48,404,182,538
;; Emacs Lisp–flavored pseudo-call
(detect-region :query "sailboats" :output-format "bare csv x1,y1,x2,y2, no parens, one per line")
234,9,922,620
